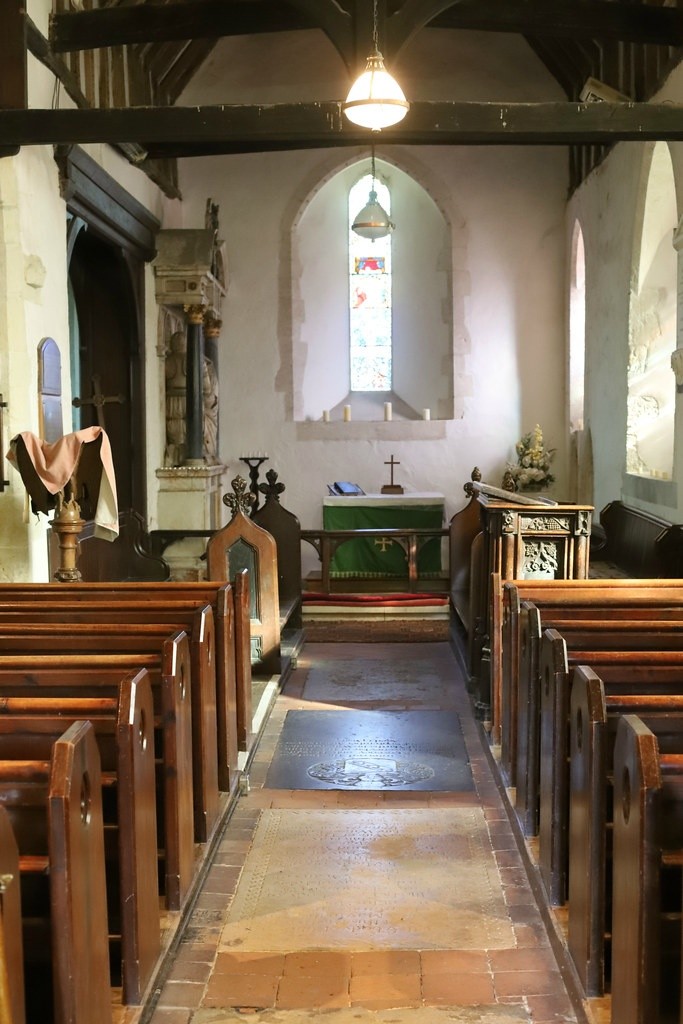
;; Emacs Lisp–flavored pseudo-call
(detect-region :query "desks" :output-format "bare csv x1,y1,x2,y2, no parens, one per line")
323,491,445,578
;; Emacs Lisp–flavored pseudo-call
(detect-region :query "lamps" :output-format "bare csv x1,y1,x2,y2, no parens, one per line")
342,0,411,133
352,133,395,242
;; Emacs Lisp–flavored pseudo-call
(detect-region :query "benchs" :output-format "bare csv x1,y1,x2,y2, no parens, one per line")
490,498,683,1024
0,567,251,1024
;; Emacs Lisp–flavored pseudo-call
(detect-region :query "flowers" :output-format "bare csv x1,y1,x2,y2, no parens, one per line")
514,424,558,493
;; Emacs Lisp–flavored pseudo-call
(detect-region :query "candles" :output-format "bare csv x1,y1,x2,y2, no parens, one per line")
384,401,392,421
323,410,329,422
423,408,430,420
343,405,351,422
241,450,267,458
